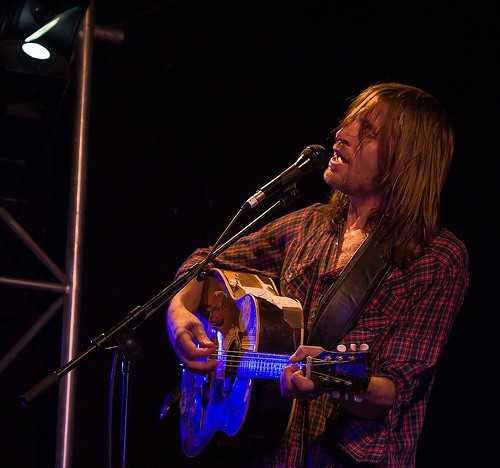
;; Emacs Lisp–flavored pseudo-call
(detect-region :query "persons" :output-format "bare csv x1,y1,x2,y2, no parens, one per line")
166,81,472,468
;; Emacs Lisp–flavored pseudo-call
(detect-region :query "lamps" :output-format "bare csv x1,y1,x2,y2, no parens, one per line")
1,2,86,60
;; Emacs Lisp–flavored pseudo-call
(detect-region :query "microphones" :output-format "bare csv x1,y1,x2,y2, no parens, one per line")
241,144,327,211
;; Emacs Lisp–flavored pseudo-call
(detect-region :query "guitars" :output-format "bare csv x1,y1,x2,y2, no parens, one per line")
179,267,376,457
